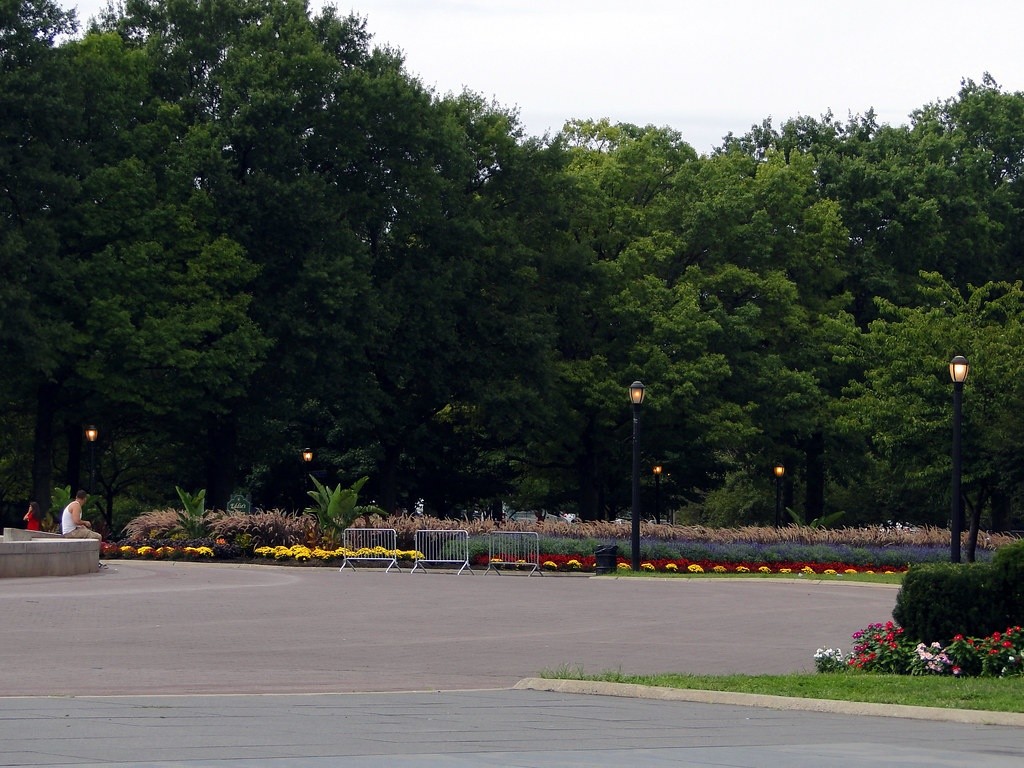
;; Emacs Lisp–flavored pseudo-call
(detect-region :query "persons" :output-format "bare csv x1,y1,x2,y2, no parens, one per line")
23,502,42,531
61,489,108,570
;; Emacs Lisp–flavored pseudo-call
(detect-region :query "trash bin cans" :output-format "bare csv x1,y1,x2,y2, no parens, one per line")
595,545,617,575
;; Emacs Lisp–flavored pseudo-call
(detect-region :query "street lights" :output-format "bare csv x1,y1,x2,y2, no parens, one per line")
628,380,647,574
85,423,98,499
652,462,662,524
773,462,785,530
950,354,968,563
302,448,313,510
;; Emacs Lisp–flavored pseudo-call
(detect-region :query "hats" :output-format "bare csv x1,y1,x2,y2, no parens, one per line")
77,490,87,498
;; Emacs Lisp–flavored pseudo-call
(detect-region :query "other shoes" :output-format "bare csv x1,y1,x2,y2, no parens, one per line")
99,562,109,569
